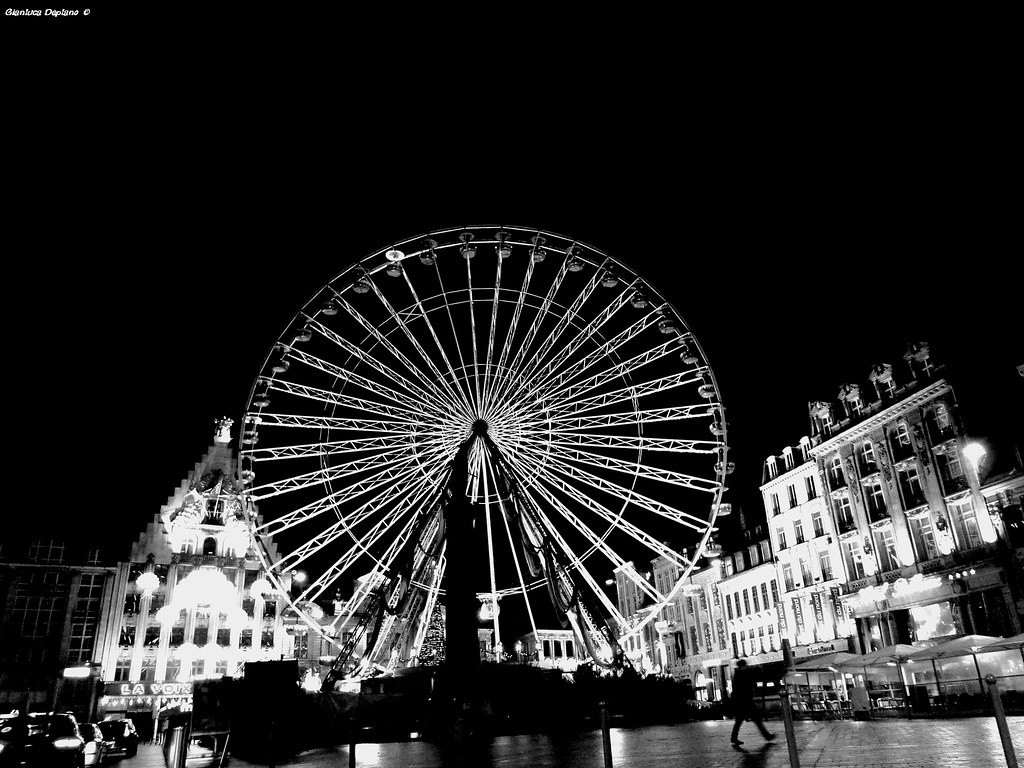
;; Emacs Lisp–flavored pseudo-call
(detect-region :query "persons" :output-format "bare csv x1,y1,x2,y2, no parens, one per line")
731,659,776,745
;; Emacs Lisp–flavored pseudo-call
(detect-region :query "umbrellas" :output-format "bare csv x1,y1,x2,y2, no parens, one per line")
787,635,1024,710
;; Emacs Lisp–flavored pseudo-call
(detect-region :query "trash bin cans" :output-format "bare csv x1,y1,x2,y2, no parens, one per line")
910,685,933,715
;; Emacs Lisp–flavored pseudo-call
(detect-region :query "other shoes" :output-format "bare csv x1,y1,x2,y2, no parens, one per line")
766,733,775,741
730,739,744,744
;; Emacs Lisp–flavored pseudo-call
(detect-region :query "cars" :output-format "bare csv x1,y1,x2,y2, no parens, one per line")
0,711,140,768
